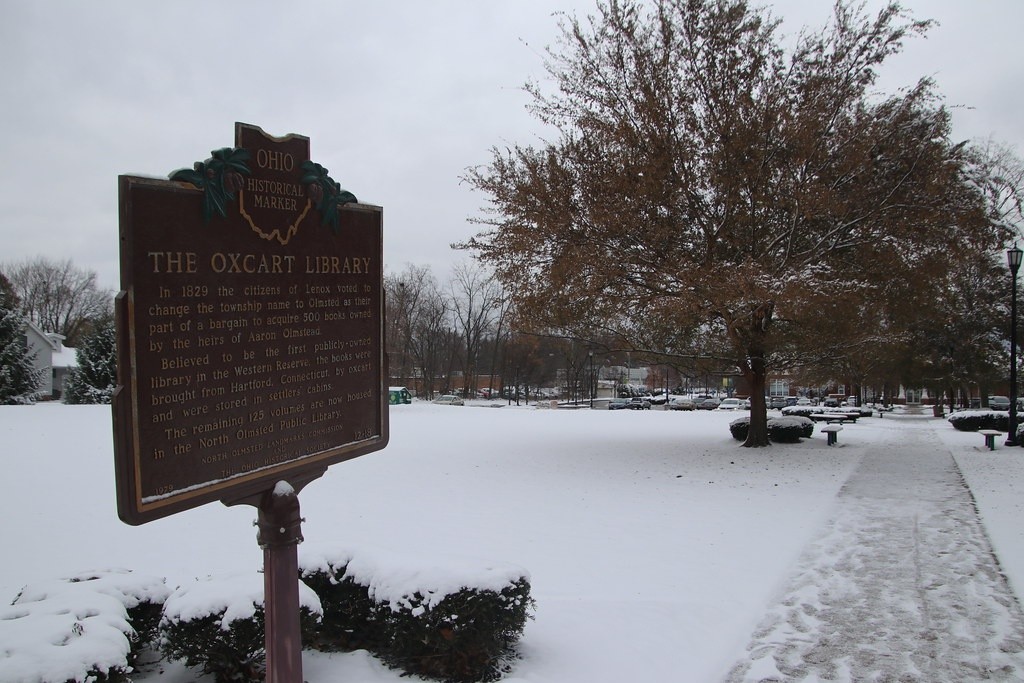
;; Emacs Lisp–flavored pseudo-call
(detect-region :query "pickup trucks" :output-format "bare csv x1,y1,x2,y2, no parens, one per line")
630,398,652,410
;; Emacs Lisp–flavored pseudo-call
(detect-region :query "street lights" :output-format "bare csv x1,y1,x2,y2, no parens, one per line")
1006,241,1024,447
589,345,594,408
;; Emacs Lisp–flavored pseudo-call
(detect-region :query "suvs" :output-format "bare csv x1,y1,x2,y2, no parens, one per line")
765,396,771,408
771,396,787,408
784,397,799,406
745,395,751,409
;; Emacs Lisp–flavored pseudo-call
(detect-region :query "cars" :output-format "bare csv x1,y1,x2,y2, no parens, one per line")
693,398,706,408
434,386,563,400
676,398,697,410
718,398,745,410
848,396,858,406
797,398,816,407
431,395,465,406
825,398,839,408
702,399,721,410
671,399,677,410
609,398,631,411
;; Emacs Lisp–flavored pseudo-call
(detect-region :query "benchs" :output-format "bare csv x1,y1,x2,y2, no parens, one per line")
979,429,1002,451
821,424,843,446
809,414,848,425
824,412,860,424
